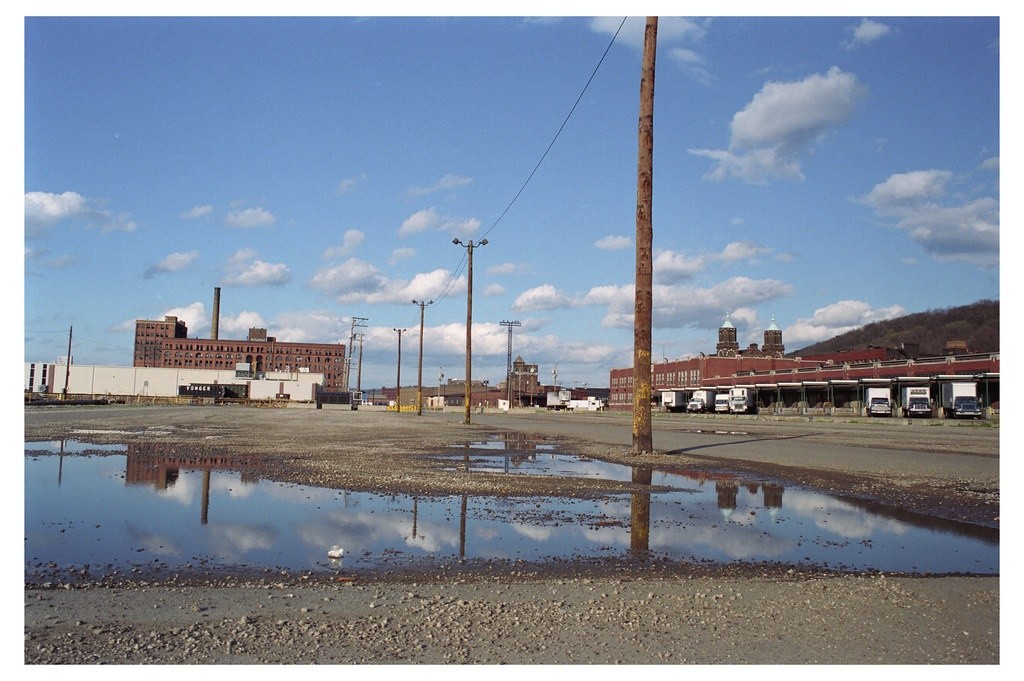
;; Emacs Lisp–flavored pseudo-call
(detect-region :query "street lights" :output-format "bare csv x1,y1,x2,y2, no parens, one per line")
393,327,407,413
452,238,489,423
411,299,434,416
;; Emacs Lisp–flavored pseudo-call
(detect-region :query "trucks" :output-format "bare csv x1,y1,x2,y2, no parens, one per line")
901,387,933,419
662,391,692,413
941,381,983,419
728,388,754,413
714,394,730,414
685,390,715,414
865,388,894,418
